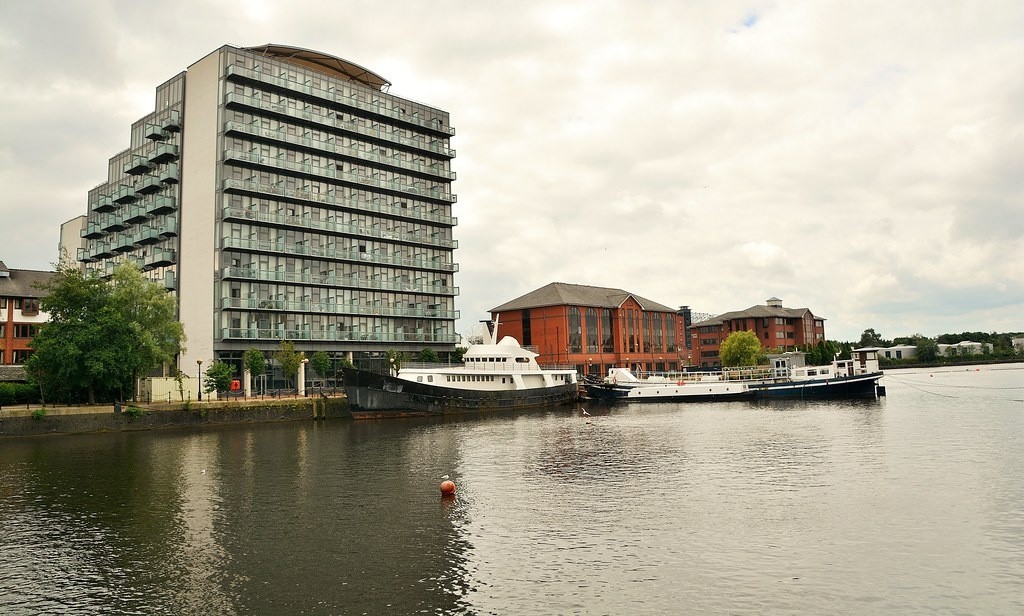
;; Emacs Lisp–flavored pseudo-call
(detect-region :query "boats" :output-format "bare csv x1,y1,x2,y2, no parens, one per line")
602,365,750,402
579,373,640,400
719,356,887,401
340,313,581,421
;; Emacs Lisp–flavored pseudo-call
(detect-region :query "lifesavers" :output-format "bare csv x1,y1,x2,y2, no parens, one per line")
677,380,683,386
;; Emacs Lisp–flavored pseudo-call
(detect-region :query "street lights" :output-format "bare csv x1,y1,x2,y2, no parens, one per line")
659,357,663,372
196,359,203,401
390,357,395,377
687,356,691,371
303,359,310,397
